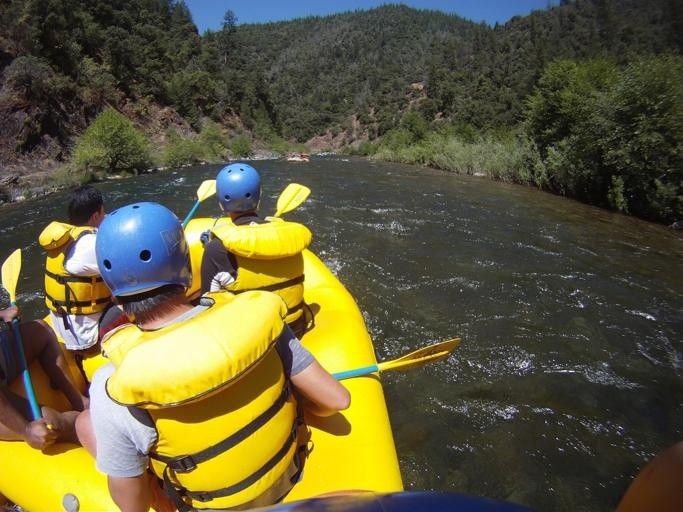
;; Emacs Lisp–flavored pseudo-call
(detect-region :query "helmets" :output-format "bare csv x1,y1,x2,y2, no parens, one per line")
95,202,193,299
216,163,261,213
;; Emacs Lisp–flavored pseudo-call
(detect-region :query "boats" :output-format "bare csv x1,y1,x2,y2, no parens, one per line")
0,217,405,512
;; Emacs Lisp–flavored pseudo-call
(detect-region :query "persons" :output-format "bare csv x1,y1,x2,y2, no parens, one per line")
0,288,90,450
190,163,313,341
239,491,531,512
39,185,128,359
74,202,351,512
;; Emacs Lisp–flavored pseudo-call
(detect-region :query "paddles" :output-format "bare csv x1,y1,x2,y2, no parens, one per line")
1,249,43,430
181,180,217,231
273,183,311,218
331,338,461,381
